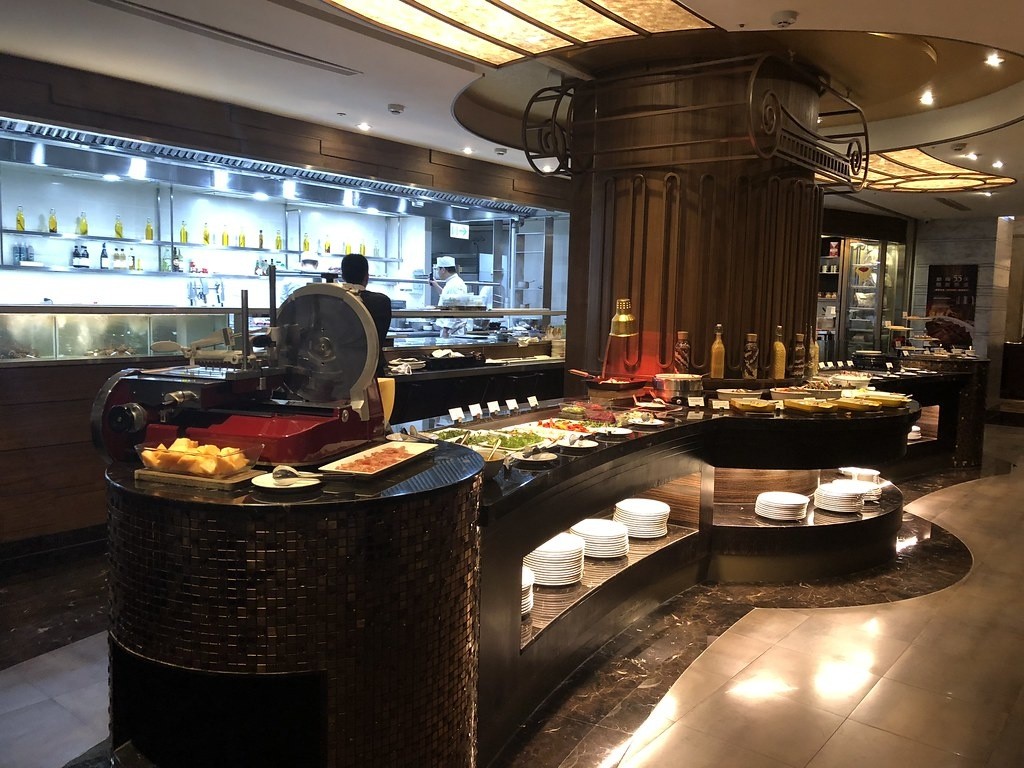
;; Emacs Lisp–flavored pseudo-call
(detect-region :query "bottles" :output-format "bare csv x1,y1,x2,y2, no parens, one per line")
674,324,819,377
12,203,383,279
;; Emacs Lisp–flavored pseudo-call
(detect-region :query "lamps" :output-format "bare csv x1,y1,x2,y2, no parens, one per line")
609,298,639,338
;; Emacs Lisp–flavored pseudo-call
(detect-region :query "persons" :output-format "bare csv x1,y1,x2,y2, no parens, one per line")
428,256,467,338
281,251,318,303
341,254,392,369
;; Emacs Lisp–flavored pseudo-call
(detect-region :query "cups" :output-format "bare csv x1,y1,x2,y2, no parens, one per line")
440,293,487,307
423,326,433,331
431,349,465,357
935,348,975,356
819,263,838,298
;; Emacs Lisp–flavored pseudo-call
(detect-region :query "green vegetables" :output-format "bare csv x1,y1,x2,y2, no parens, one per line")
436,427,543,448
553,418,613,427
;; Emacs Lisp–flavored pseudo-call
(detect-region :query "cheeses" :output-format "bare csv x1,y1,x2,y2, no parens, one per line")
141,438,247,473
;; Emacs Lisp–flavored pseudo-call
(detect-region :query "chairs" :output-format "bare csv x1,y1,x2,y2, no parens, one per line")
394,367,565,420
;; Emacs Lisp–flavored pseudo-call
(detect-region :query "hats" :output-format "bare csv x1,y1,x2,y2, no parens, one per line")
436,256,456,267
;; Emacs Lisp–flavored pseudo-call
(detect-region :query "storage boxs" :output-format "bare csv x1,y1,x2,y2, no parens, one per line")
422,352,476,371
0,303,230,366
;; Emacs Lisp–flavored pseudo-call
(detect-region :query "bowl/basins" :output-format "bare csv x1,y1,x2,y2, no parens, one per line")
558,401,633,423
717,376,870,400
476,449,505,480
133,436,266,478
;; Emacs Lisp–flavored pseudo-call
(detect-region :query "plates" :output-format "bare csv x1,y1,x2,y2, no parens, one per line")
521,497,671,617
908,425,922,439
754,466,882,521
754,500,881,527
388,358,426,371
636,403,666,408
520,536,665,649
729,390,913,410
433,417,667,461
813,351,979,382
252,431,440,488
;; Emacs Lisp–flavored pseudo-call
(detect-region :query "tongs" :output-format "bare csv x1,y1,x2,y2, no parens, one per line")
400,425,438,444
272,465,355,486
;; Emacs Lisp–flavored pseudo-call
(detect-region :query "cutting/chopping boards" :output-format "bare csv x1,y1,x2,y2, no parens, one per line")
133,465,267,491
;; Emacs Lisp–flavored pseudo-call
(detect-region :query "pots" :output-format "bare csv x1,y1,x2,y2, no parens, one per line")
569,370,647,389
391,300,508,331
651,372,703,406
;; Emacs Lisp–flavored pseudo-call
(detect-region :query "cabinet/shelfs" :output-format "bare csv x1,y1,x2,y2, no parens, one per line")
512,216,553,327
478,371,975,768
839,237,900,361
0,230,400,287
817,236,842,336
